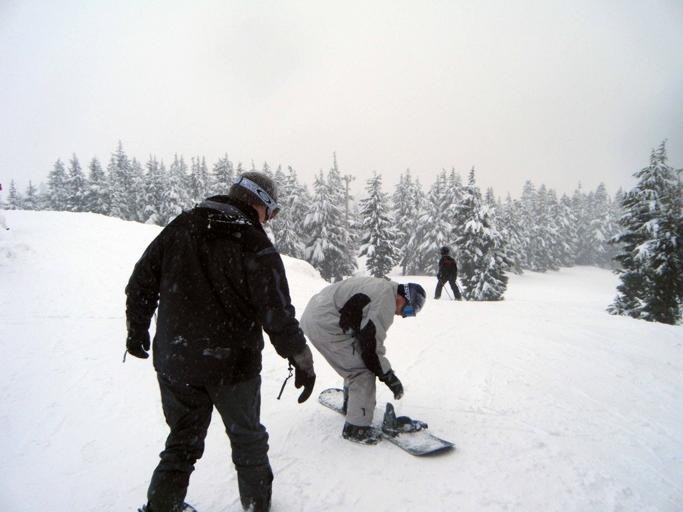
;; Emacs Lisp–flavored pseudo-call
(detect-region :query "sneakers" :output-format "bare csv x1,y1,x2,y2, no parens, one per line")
342,422,377,443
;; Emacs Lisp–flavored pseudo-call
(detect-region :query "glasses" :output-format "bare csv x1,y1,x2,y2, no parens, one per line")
402,283,417,318
236,176,281,222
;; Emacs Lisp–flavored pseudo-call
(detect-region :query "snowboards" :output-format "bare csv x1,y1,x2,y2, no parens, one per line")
318,388,456,458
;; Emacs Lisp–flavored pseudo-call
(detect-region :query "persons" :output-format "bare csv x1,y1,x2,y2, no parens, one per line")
124,171,317,512
299,274,427,446
433,246,462,301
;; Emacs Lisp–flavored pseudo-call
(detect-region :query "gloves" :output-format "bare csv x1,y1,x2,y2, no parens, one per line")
291,345,315,403
382,370,404,400
123,311,150,359
